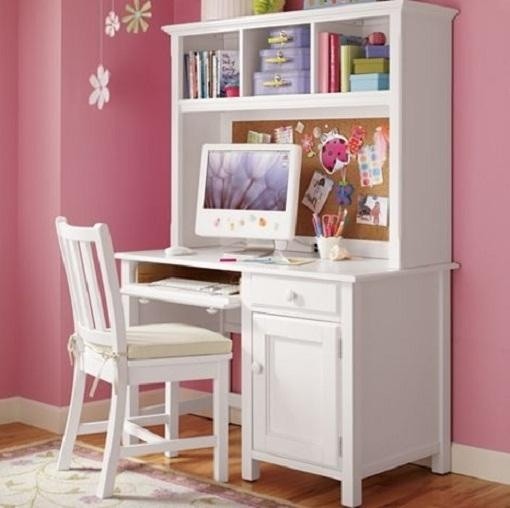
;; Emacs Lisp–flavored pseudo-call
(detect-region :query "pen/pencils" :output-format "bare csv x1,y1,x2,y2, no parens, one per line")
219,258,236,262
312,205,349,238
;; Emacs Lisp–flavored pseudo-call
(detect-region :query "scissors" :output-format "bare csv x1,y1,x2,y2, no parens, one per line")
320,213,339,236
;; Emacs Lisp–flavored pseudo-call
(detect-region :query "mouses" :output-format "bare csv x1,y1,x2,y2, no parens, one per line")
165,246,192,256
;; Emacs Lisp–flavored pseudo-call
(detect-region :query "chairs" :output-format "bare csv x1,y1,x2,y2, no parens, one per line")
53,215,234,500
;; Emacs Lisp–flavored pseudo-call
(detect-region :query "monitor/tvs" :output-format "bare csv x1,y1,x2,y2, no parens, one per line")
195,143,303,264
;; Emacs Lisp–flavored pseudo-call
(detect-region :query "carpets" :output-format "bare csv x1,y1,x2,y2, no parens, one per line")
1,437,310,508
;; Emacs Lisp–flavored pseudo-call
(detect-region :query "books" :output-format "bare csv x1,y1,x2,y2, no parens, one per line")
339,42,365,93
181,46,238,99
330,32,341,92
319,29,330,92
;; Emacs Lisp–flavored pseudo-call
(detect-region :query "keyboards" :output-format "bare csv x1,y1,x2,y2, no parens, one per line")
148,276,240,295
